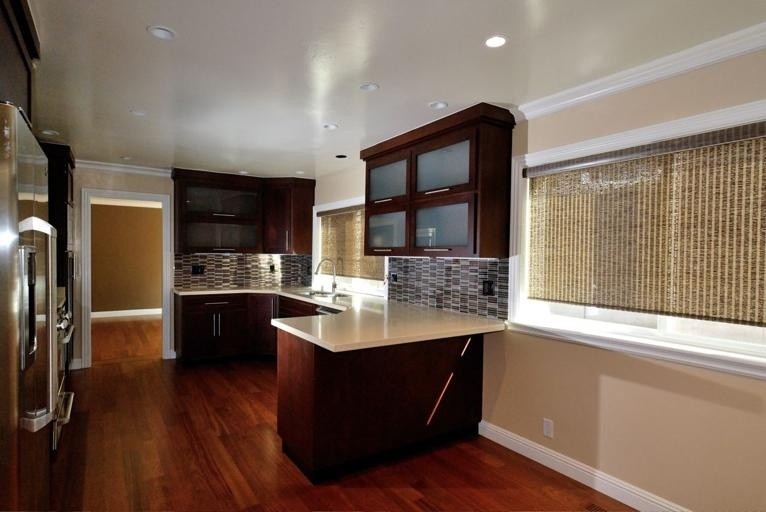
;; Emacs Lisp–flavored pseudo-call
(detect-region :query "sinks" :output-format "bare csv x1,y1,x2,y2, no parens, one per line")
296,291,351,298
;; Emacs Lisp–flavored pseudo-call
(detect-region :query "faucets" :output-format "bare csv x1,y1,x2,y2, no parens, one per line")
314,259,337,293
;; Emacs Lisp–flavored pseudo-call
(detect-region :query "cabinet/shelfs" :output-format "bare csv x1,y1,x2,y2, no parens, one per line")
260,175,317,259
357,99,515,263
243,292,279,362
276,295,320,320
170,167,265,253
171,292,252,362
31,138,76,378
